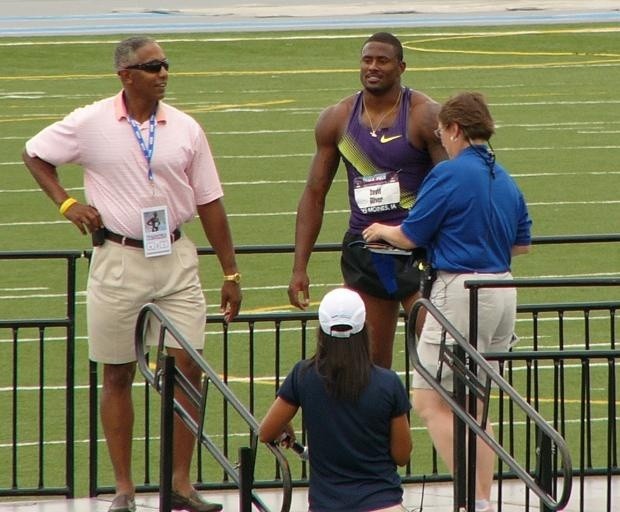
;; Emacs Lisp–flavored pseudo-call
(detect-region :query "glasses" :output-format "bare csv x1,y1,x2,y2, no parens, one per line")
126,57,169,73
434,122,449,139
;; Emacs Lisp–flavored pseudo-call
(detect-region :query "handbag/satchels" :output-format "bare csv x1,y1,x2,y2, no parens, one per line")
336,228,421,300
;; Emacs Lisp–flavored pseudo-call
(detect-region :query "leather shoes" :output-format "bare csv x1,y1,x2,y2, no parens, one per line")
108,496,136,512
170,490,222,511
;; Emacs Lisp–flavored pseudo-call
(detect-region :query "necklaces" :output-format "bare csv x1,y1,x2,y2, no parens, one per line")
361,86,402,139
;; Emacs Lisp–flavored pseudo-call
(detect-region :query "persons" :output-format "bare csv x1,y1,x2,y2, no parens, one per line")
17,32,246,512
256,284,413,512
146,212,161,231
285,31,450,370
362,90,535,512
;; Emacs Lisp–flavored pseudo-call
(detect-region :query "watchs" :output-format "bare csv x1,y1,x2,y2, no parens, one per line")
223,272,242,285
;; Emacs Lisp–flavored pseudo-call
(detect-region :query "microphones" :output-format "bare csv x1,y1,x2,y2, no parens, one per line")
280,432,309,459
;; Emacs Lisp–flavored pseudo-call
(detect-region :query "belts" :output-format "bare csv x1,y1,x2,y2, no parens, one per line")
106,230,180,248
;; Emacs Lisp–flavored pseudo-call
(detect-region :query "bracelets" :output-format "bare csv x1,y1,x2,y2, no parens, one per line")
58,195,76,217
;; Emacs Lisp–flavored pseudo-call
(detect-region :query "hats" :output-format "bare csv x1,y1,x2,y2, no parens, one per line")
318,288,366,339
